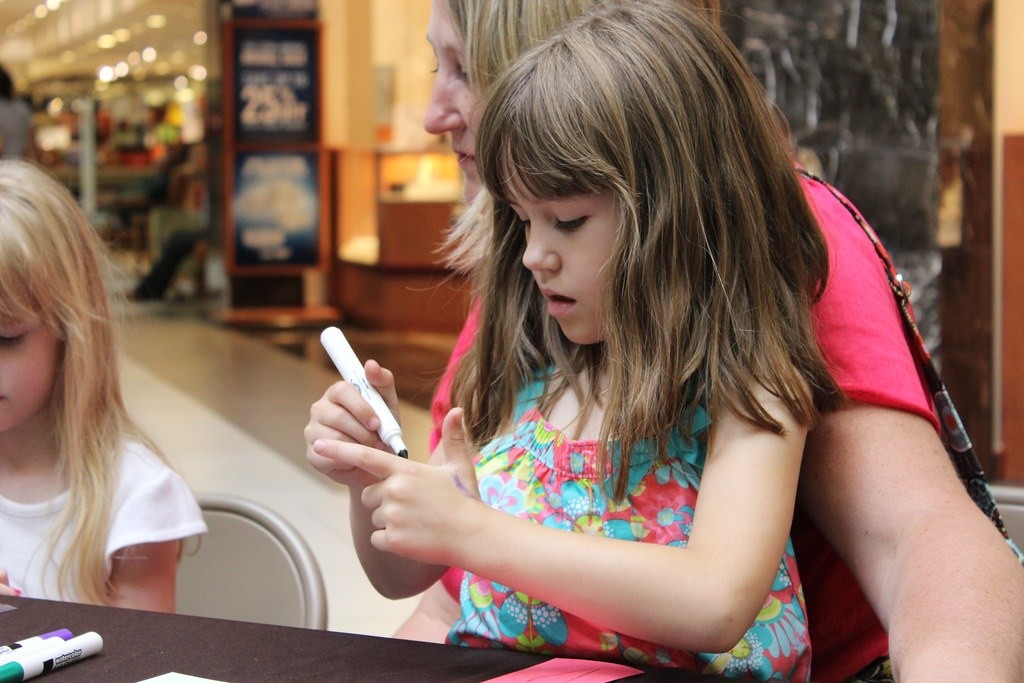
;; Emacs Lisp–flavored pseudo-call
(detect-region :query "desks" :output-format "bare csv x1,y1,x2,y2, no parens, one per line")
0,593,751,683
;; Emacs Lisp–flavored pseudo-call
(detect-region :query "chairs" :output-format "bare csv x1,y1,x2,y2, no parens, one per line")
174,493,329,631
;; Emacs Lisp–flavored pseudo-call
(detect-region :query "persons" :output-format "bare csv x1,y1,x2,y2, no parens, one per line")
1,66,33,159
299,0,833,682
1,156,209,622
374,0,1023,683
119,144,205,302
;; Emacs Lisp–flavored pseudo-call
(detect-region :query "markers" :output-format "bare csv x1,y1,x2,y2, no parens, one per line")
0,614,75,660
319,326,409,463
0,631,105,682
0,637,69,669
